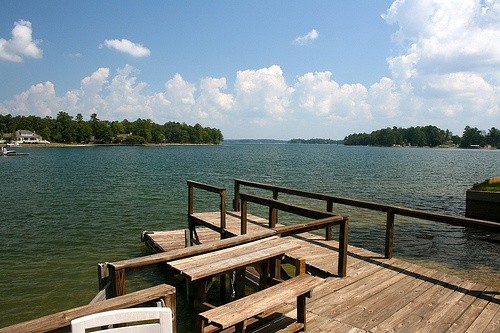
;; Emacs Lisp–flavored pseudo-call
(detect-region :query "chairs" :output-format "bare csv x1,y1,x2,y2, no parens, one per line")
70,306,174,333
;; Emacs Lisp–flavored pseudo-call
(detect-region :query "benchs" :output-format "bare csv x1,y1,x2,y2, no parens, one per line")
199,273,321,333
